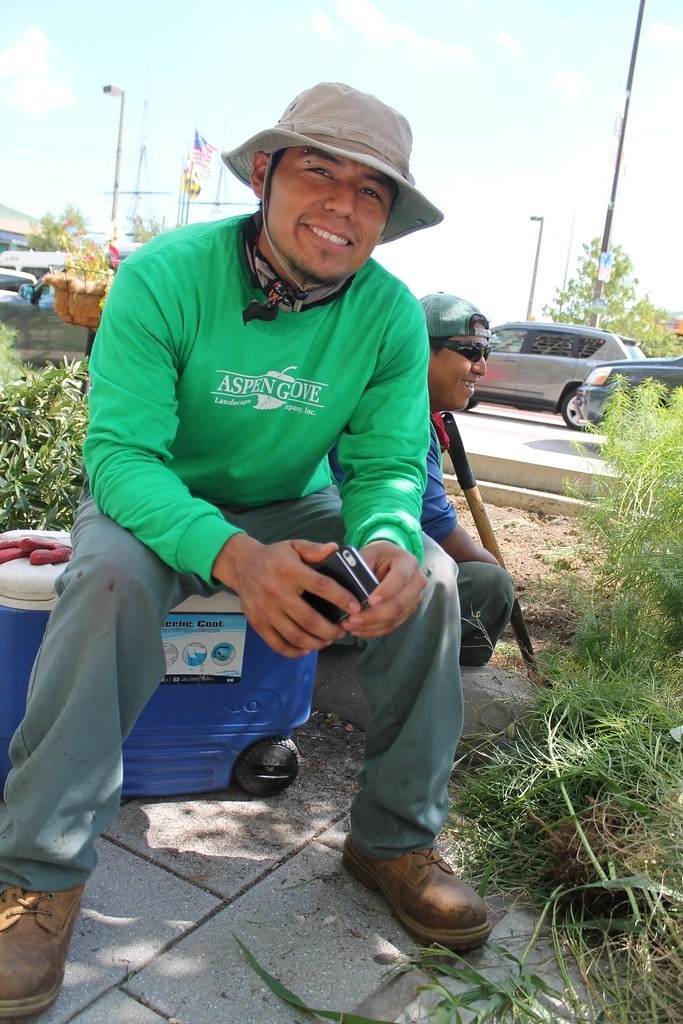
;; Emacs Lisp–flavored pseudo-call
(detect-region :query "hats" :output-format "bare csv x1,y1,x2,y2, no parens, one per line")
419,292,491,338
221,82,443,244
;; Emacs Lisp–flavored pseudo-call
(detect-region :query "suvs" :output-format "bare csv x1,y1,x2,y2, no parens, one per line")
456,320,647,431
576,355,683,429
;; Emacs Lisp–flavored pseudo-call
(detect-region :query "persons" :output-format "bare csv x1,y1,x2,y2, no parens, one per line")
0,82,495,1019
329,290,514,666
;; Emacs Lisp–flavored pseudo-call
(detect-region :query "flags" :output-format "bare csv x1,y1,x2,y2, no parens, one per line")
179,131,216,200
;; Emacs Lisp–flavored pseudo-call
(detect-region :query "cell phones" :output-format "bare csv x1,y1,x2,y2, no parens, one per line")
298,545,383,625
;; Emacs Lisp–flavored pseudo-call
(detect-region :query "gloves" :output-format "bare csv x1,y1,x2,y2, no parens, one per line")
0,534,73,565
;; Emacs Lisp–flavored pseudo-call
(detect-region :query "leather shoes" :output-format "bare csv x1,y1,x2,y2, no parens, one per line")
344,831,492,952
0,880,85,1018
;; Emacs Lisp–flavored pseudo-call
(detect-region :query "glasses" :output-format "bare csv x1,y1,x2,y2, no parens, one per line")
430,340,491,362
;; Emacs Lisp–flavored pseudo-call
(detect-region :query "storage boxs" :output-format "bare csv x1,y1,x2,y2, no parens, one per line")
0,529,314,799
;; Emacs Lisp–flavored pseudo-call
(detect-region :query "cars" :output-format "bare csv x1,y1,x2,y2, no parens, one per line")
0,242,144,305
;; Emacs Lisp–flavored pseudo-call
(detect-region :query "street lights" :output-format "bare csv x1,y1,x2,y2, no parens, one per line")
525,216,545,321
101,83,125,246
